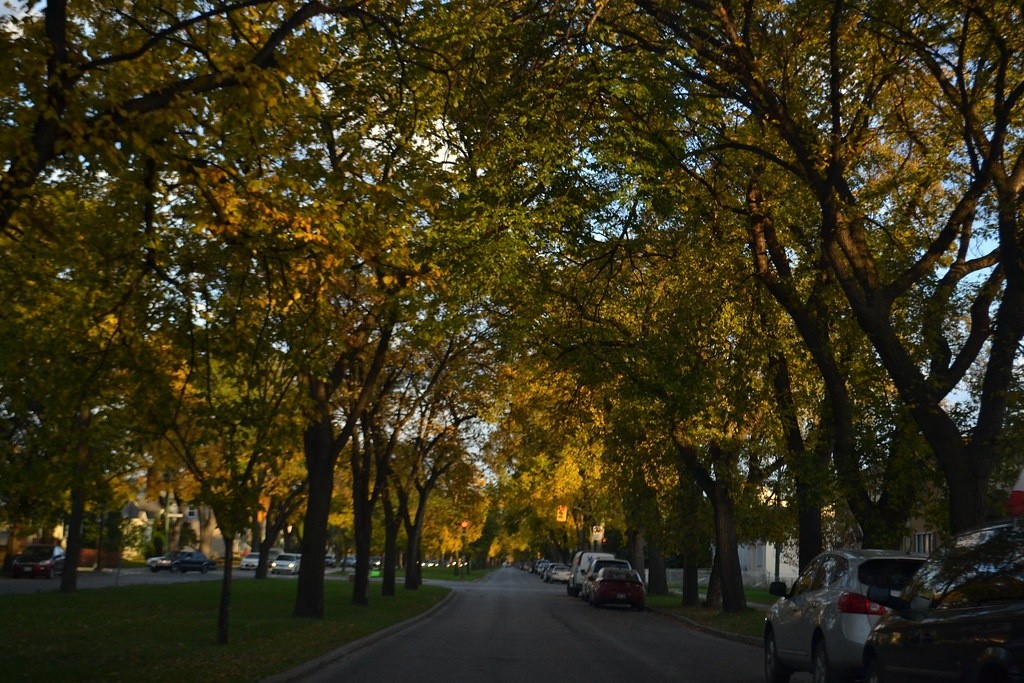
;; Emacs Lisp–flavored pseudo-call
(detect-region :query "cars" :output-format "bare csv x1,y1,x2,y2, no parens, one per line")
529,561,537,573
370,557,382,567
550,566,572,584
269,549,285,566
763,546,927,683
541,563,549,579
544,563,566,582
325,555,336,566
271,554,302,575
860,517,1024,683
520,562,530,570
340,557,357,566
537,563,547,574
240,553,260,569
13,544,66,578
147,550,218,574
534,560,550,574
580,557,632,602
588,567,645,609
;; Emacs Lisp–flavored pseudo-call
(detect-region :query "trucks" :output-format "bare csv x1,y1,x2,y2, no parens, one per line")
566,551,616,597
